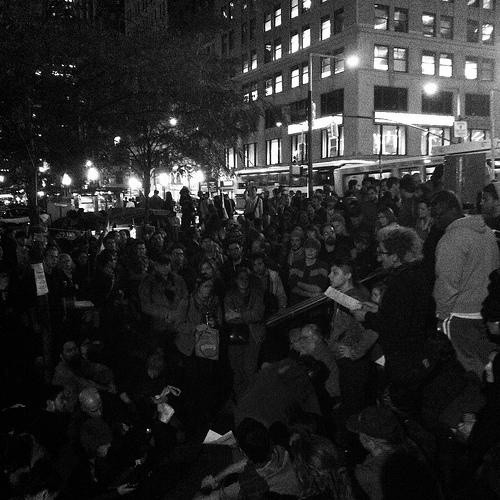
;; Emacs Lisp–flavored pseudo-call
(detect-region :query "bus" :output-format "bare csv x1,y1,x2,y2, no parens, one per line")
333,139,500,206
216,158,378,212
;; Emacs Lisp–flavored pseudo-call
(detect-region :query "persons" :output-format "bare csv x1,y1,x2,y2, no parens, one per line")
428,162,444,182
486,158,498,183
1,172,500,499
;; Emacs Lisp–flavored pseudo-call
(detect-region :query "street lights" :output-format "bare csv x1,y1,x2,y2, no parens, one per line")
307,52,360,199
423,82,461,120
